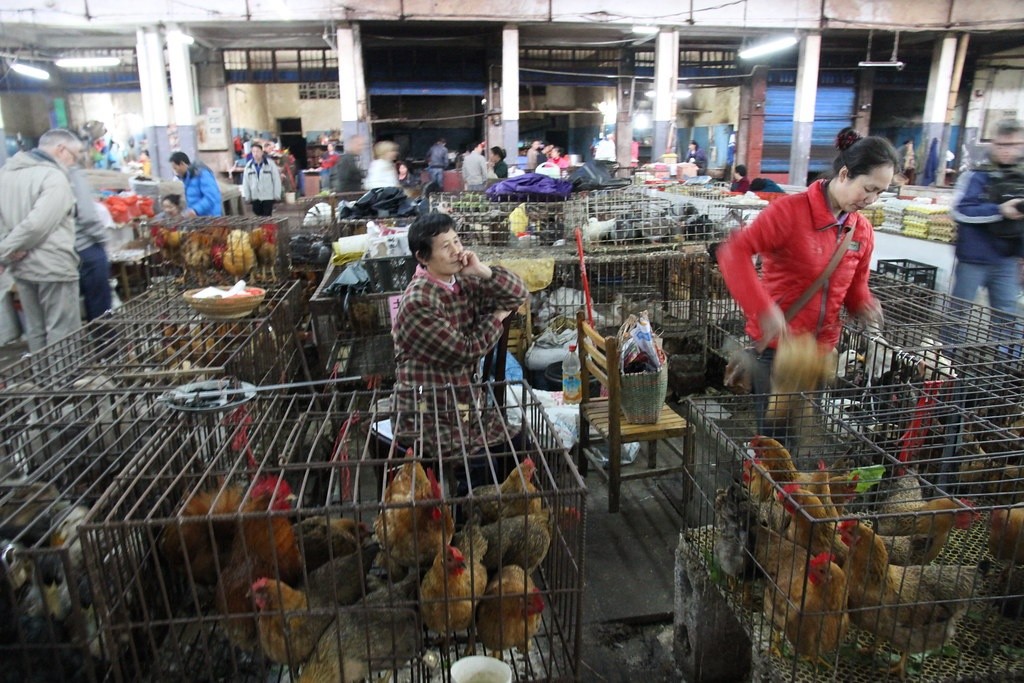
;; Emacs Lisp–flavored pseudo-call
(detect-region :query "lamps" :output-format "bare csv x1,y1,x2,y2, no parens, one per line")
858,4,905,70
739,0,800,60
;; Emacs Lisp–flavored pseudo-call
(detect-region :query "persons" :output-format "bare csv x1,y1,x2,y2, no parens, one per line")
896,137,918,186
169,151,224,225
590,132,640,175
328,135,365,192
234,130,294,190
320,143,341,189
685,140,708,177
81,120,153,182
0,128,115,393
454,139,568,196
715,128,899,460
939,115,1024,358
730,165,750,192
242,144,281,219
391,212,531,485
750,178,787,193
363,140,402,190
150,194,186,223
425,139,449,191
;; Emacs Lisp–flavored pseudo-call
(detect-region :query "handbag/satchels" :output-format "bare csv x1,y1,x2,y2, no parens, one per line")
568,159,632,192
524,328,593,370
723,347,755,395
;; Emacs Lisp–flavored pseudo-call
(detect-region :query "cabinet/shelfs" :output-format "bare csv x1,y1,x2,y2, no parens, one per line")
639,144,652,158
307,143,344,167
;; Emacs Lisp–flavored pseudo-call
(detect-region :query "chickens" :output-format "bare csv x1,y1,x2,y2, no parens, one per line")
715,313,1023,666
151,220,279,287
166,448,561,683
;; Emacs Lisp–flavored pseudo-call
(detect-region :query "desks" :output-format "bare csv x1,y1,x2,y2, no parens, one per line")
110,245,160,297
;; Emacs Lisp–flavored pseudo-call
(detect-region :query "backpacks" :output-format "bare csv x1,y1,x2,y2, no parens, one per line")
972,164,1024,258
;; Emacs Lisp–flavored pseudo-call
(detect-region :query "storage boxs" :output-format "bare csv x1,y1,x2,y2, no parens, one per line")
878,258,938,324
363,250,418,294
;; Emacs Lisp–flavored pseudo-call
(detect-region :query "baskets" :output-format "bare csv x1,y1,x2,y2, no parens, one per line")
614,311,669,423
183,285,266,319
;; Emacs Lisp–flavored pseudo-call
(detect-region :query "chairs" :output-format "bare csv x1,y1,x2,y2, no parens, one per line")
577,309,697,514
370,307,513,510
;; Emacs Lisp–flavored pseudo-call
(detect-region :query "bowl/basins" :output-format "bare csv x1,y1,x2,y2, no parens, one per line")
184,285,267,320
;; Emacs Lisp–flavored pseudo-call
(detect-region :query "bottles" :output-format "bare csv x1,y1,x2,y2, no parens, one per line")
562,345,582,405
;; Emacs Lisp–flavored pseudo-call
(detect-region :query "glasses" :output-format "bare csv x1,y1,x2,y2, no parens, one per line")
63,147,81,164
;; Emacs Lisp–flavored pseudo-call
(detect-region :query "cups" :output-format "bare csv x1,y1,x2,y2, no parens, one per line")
451,655,512,683
285,192,295,203
